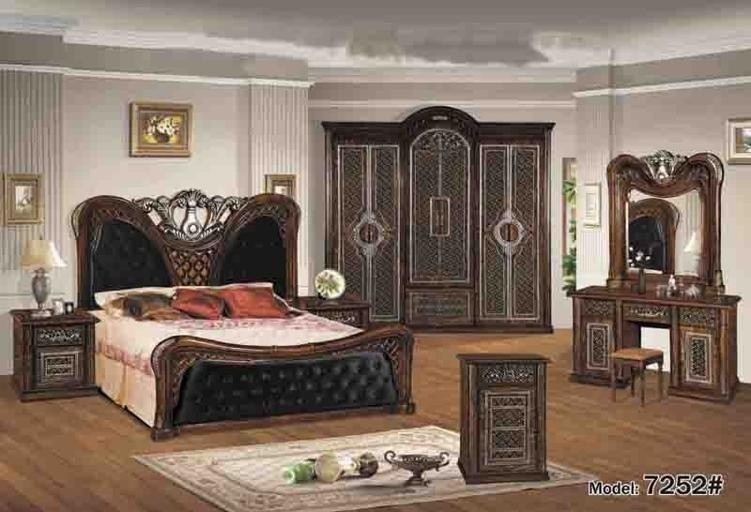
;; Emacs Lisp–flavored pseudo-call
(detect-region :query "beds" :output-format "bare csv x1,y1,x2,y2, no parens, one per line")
69,186,420,444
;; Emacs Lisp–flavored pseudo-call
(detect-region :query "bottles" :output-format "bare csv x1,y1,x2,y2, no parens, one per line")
279,457,320,485
666,275,679,300
712,269,727,304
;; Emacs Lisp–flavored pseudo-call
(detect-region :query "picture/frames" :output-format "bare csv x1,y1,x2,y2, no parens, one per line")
2,170,48,228
123,99,198,162
264,173,299,204
578,180,603,229
725,112,751,167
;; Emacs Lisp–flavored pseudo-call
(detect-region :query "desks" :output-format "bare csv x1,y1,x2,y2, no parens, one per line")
567,284,743,407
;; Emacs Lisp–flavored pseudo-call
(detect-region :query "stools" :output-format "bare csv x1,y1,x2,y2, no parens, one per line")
606,346,667,409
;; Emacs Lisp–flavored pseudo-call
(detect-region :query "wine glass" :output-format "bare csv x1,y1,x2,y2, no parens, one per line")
315,452,378,482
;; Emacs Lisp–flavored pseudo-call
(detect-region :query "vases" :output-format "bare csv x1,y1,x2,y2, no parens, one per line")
636,269,649,295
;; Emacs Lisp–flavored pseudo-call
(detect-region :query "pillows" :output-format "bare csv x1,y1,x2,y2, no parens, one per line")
168,281,227,322
93,284,177,322
112,290,183,325
173,277,292,309
202,285,294,321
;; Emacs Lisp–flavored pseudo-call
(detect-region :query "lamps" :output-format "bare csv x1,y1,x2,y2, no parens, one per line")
15,238,71,317
682,227,703,276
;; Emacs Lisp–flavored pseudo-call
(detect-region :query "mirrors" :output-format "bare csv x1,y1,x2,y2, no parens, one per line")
605,147,727,301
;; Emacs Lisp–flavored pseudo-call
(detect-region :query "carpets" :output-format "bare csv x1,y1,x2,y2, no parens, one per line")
127,420,609,511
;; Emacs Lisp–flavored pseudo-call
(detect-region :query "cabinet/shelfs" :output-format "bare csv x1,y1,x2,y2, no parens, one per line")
401,283,477,329
477,125,547,330
453,350,553,485
405,105,477,287
328,128,406,327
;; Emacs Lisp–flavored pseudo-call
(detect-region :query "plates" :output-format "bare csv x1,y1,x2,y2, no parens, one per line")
315,269,347,300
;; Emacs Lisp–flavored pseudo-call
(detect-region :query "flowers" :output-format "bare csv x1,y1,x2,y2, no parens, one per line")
625,243,653,271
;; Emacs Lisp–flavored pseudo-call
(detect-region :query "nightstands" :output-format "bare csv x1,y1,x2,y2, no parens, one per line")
7,304,101,402
291,290,374,332
